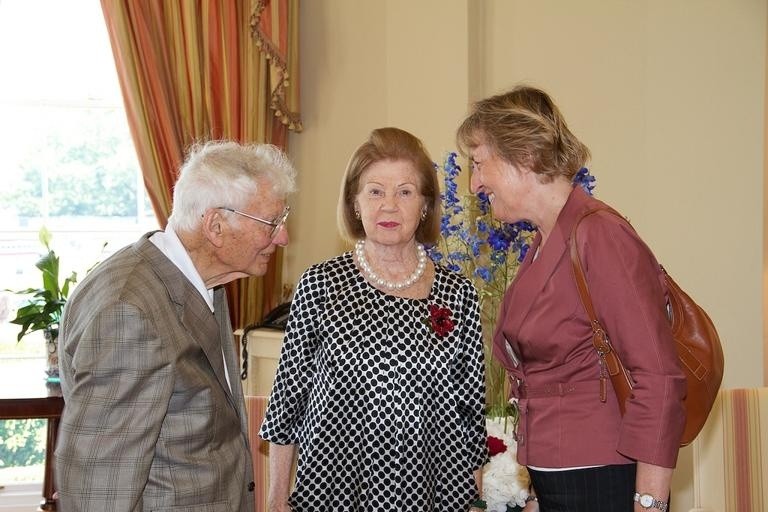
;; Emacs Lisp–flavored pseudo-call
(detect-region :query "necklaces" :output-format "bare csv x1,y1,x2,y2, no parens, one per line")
355,236,428,292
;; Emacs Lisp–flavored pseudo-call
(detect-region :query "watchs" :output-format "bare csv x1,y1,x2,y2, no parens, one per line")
632,490,670,512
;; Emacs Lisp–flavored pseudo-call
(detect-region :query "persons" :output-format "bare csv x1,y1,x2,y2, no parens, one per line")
455,85,726,512
258,124,494,511
46,141,296,512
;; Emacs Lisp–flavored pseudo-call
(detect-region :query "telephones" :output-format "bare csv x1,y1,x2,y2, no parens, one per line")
263,301,292,329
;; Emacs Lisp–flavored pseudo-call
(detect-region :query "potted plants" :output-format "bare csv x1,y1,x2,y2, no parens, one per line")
0,226,109,382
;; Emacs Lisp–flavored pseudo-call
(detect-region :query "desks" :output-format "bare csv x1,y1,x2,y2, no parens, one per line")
0,354,65,512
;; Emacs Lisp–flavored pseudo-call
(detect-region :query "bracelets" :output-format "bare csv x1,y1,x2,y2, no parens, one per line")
524,493,540,505
469,496,490,510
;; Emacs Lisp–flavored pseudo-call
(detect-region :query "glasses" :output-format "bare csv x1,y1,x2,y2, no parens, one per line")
200,204,292,238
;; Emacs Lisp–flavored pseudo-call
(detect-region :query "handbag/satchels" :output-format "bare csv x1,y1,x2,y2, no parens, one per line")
567,206,725,448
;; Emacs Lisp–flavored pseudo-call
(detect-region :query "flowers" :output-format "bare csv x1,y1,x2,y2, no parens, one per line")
425,152,598,434
